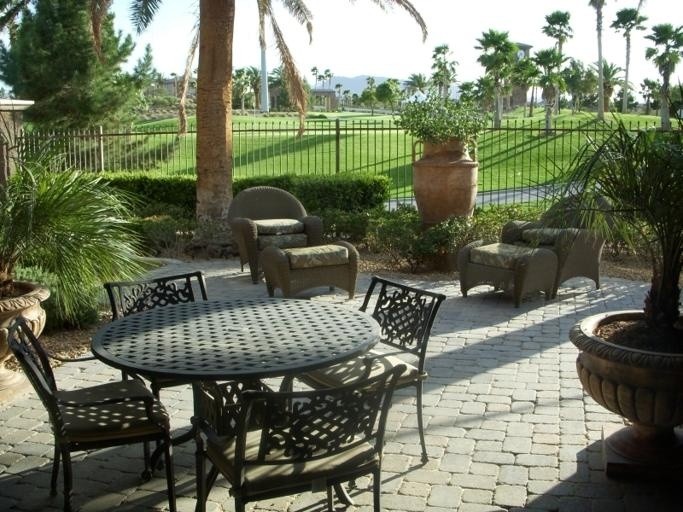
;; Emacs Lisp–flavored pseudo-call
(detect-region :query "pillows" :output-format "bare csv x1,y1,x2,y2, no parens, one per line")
522,228,562,245
254,218,304,235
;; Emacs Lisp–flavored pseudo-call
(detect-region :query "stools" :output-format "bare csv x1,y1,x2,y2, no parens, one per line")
457,240,558,308
261,241,360,299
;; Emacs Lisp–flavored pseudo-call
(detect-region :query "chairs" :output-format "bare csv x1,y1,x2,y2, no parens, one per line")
228,186,323,284
502,193,614,299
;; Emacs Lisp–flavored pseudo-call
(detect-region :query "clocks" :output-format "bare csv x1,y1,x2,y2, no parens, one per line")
517,50,525,58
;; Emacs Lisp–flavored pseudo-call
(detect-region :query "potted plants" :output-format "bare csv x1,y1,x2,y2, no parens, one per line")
0,129,158,357
560,128,683,463
390,91,492,230
